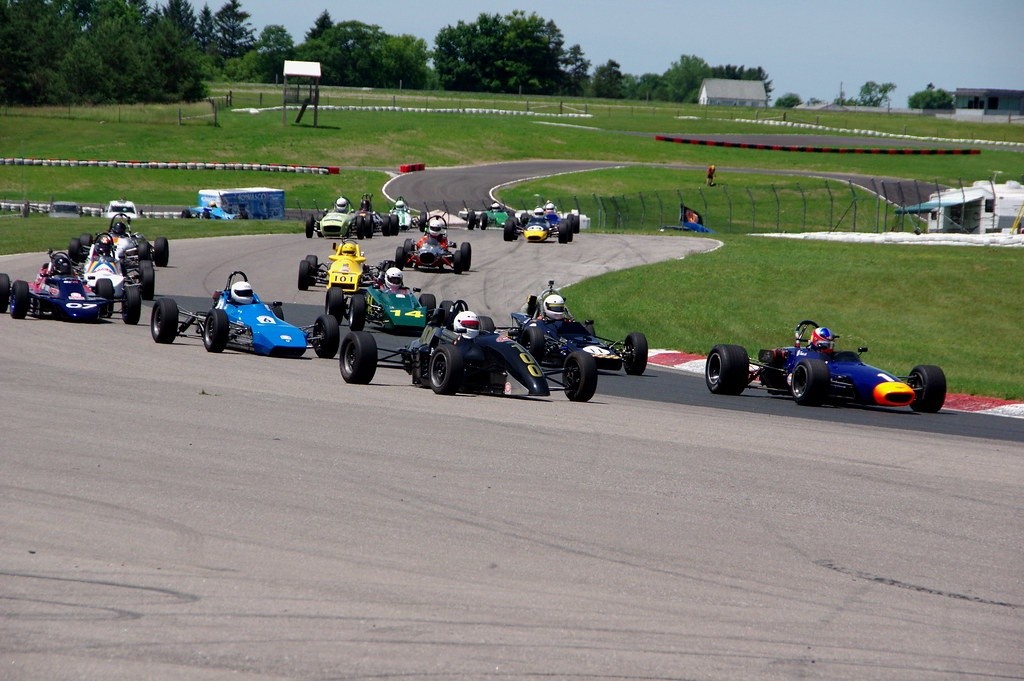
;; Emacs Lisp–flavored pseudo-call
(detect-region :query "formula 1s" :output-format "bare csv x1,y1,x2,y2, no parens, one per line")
0,213,169,327
337,299,601,402
296,193,582,357
503,278,651,376
152,271,342,359
705,318,950,414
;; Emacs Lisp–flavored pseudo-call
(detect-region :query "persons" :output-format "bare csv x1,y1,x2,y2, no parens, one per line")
230,281,254,304
390,200,410,213
492,203,501,212
109,223,134,249
417,220,449,250
342,243,355,255
538,294,571,321
706,165,715,186
336,196,348,213
546,203,556,213
34,255,91,295
381,267,411,294
454,311,480,339
361,200,370,211
811,327,834,355
210,200,217,207
84,237,120,272
533,207,545,218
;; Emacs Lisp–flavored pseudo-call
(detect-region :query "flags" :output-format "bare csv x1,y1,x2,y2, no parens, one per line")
679,204,704,225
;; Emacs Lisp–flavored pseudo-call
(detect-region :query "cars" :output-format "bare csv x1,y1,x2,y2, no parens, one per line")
105,200,139,219
49,200,81,218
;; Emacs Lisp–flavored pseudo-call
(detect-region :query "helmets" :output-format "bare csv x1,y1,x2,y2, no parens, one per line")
385,267,403,290
342,243,355,256
336,198,347,212
454,311,481,339
546,203,555,213
810,326,833,356
395,201,404,210
534,208,544,218
361,200,370,208
429,220,441,236
114,222,126,234
492,203,502,212
542,294,565,320
95,236,111,255
231,280,254,304
53,255,71,275
